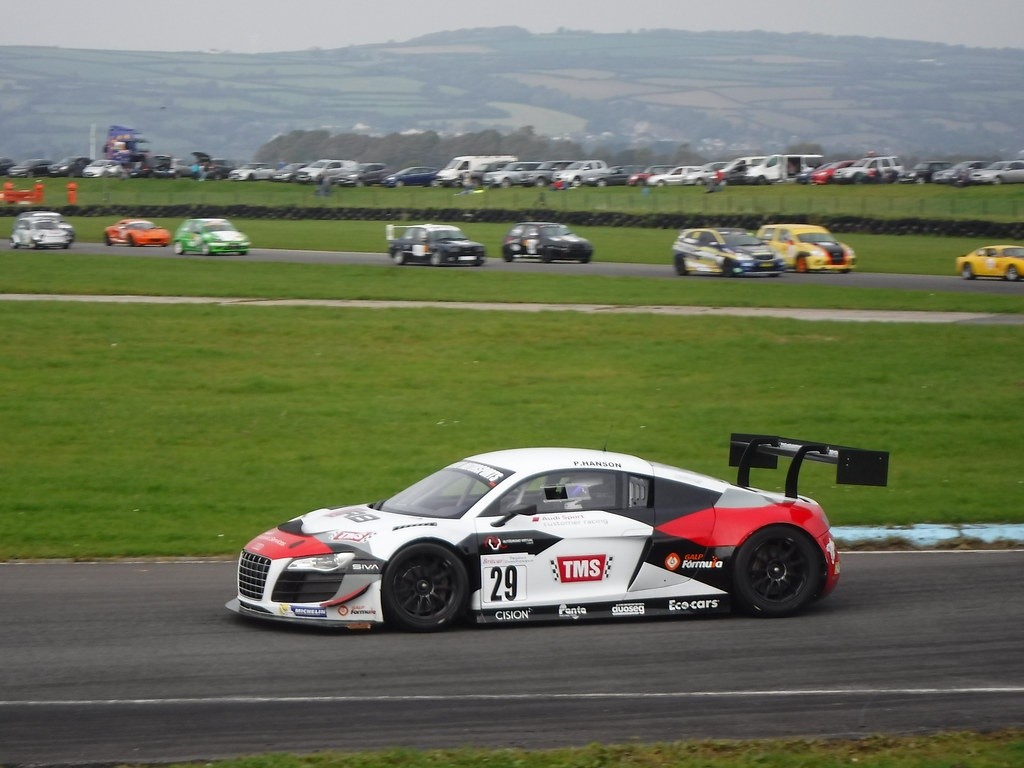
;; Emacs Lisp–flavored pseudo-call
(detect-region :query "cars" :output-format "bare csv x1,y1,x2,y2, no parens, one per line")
954,243,1024,282
384,221,487,266
8,210,77,250
670,222,862,281
173,217,252,256
0,148,1024,191
501,220,595,265
223,428,891,634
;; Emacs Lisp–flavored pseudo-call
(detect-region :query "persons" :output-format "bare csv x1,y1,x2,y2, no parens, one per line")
885,169,898,182
192,160,199,178
554,179,565,190
704,170,721,193
198,163,208,180
462,173,472,191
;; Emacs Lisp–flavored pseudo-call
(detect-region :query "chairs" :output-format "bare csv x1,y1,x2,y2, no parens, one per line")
581,484,616,508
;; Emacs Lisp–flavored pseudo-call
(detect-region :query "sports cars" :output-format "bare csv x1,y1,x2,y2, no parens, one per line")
103,216,172,249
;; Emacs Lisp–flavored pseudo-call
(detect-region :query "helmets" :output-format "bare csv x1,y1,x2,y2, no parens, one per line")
564,485,593,510
